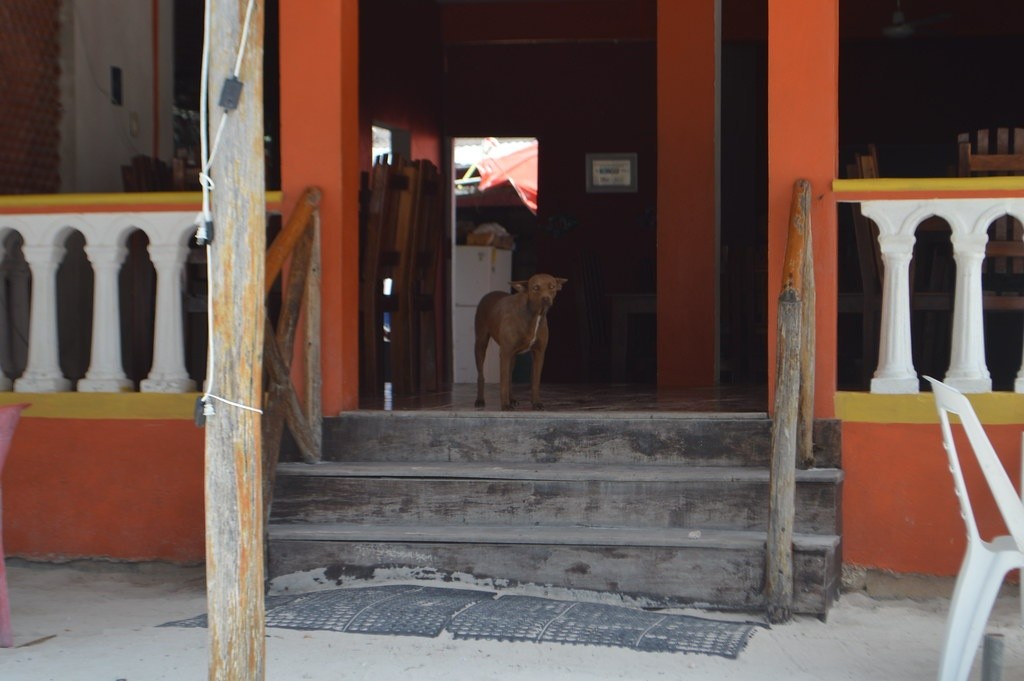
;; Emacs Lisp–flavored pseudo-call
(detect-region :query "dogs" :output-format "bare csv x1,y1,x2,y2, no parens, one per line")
473,273,568,410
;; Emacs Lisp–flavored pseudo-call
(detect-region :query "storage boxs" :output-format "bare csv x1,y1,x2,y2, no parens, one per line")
467,233,513,250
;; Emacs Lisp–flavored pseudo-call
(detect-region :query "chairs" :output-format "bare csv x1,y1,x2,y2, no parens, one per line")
122,154,208,392
358,154,445,402
841,145,1023,389
578,250,629,390
922,374,1024,681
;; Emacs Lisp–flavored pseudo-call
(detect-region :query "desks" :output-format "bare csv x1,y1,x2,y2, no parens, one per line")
604,290,743,386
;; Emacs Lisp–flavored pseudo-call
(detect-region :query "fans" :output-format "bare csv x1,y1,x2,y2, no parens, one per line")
883,0,955,42
